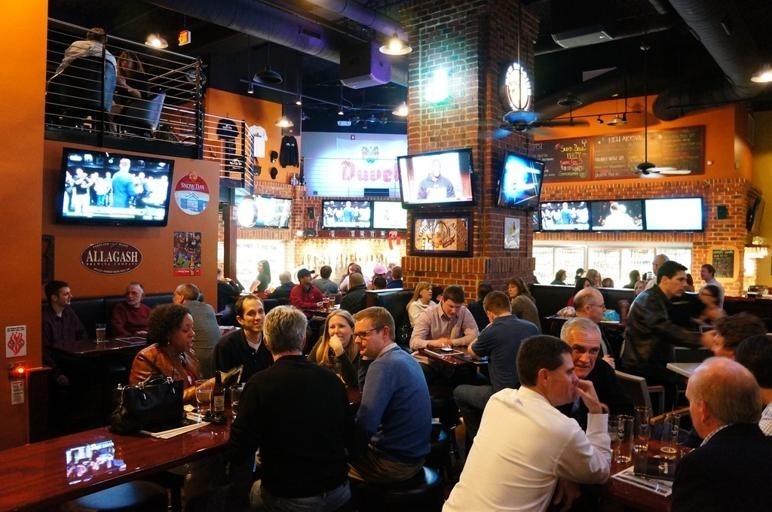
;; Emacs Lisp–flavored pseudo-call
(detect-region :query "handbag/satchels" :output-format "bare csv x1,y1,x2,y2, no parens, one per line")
109,378,187,435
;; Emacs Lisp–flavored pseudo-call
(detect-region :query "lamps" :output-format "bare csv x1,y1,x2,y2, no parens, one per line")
247,82,254,94
596,72,656,175
377,23,413,57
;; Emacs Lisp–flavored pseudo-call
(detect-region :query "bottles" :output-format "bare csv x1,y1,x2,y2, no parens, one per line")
188,255,196,276
618,298,630,323
210,370,227,425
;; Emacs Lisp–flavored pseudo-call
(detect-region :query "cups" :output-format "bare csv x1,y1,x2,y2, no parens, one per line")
289,173,299,185
228,384,244,421
194,380,212,415
646,272,654,282
322,296,336,312
95,322,107,344
614,406,682,464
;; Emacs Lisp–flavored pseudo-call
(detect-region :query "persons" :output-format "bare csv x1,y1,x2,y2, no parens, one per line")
543,200,645,230
322,201,370,227
338,306,435,486
65,439,127,485
55,27,120,136
42,279,90,395
416,159,456,200
109,49,151,131
125,253,772,436
668,355,771,511
206,303,358,511
59,155,168,220
440,333,615,511
110,280,156,371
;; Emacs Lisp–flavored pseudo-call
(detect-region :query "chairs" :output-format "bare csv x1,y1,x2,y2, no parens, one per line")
46,57,167,137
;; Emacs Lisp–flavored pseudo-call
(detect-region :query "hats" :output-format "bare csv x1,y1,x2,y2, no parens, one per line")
297,269,315,277
374,266,385,275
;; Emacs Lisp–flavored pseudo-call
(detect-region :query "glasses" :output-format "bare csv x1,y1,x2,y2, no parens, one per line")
353,328,378,340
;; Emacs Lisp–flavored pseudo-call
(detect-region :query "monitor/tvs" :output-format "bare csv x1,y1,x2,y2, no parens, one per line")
397,147,475,209
372,199,407,230
749,198,765,235
53,146,175,228
540,201,592,232
531,206,540,232
322,200,372,229
645,196,705,232
254,196,292,228
592,199,645,232
494,150,546,211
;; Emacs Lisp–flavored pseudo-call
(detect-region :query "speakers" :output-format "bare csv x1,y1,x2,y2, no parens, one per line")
339,40,391,89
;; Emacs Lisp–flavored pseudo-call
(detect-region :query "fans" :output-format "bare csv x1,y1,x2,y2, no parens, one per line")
456,0,589,141
